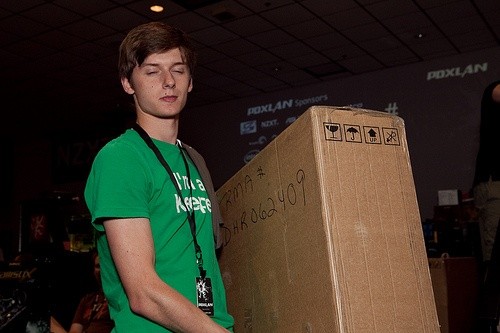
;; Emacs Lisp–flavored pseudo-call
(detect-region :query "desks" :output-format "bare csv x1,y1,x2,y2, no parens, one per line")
428,255,479,333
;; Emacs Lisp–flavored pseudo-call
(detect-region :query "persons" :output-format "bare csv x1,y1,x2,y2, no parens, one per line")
0,244,115,333
83,20,235,332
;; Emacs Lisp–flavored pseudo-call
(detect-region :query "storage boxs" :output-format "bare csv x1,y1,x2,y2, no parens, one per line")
213,105,441,333
